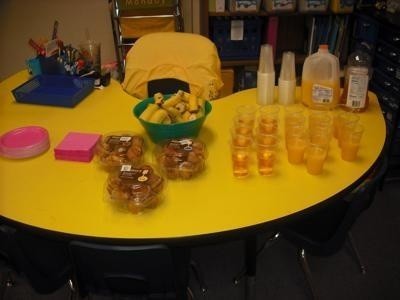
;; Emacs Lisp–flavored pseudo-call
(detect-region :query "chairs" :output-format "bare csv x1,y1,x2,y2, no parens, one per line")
234,166,390,285
124,32,221,105
66,240,207,299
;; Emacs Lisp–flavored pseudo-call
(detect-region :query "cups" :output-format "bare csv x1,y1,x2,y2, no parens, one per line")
256,42,297,107
230,103,364,176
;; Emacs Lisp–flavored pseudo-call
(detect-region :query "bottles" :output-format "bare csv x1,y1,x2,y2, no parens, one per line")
339,41,374,113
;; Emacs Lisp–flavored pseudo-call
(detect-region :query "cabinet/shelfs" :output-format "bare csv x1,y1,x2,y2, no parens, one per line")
178,0,400,94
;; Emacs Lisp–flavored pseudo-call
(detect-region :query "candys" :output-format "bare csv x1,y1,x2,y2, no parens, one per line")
97,135,206,214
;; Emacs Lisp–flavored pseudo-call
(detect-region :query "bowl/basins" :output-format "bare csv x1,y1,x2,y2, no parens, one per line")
131,94,212,145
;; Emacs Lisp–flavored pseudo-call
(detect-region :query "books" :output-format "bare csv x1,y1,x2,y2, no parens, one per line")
302,17,352,60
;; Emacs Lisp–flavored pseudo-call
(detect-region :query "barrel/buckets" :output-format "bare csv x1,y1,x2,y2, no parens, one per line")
302,44,341,110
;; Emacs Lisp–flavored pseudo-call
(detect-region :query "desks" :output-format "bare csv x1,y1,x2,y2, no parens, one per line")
1,66,388,241
